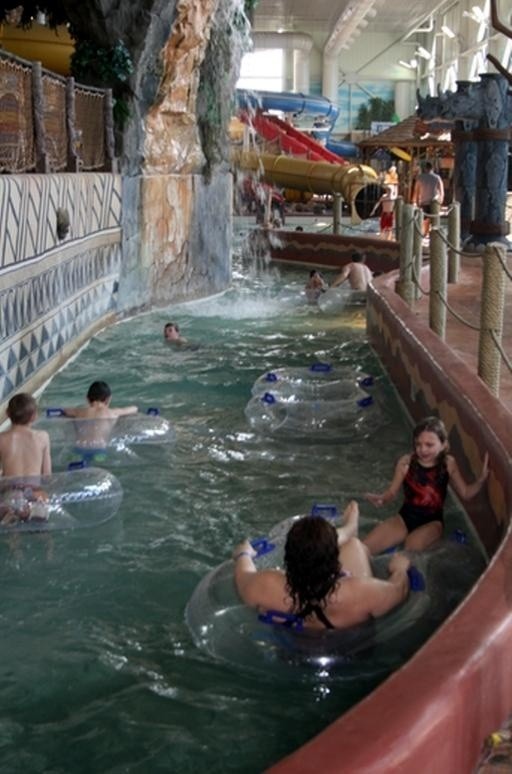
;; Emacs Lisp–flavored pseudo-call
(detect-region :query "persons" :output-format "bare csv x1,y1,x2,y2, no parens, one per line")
163,320,189,347
0,394,52,526
352,415,493,562
232,499,413,645
332,249,376,302
414,160,444,237
303,268,326,296
60,380,141,469
370,184,402,239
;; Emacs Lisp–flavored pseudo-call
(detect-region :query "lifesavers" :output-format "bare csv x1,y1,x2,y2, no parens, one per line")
266,503,465,582
1,466,124,531
32,408,175,469
186,530,431,684
247,361,387,437
319,288,368,313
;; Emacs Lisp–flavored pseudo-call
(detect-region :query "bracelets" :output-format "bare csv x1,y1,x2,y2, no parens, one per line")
234,552,252,559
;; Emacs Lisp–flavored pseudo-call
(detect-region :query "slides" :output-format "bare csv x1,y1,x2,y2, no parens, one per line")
232,88,387,226
